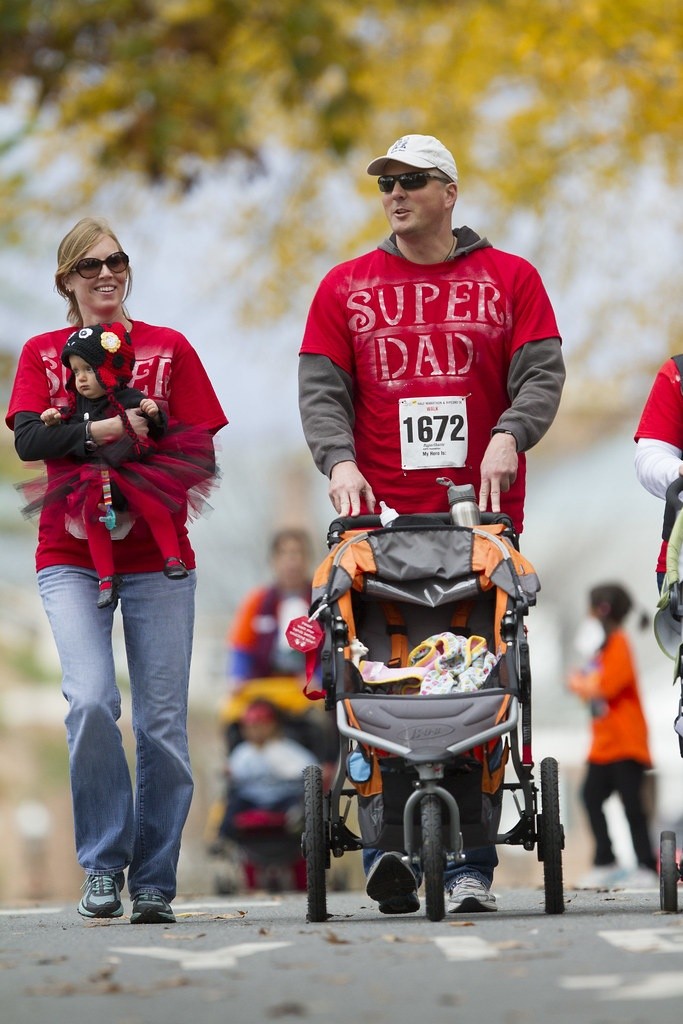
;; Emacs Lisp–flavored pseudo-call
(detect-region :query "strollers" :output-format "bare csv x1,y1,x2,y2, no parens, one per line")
286,512,565,922
653,477,683,912
207,679,339,893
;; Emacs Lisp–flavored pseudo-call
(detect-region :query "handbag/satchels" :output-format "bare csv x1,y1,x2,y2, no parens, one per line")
406,631,497,695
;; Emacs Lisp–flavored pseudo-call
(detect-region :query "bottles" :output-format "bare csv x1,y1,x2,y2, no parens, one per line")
380,500,399,528
436,477,480,528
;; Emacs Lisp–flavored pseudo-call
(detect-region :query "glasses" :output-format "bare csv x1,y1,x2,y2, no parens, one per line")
70,252,130,280
378,172,451,192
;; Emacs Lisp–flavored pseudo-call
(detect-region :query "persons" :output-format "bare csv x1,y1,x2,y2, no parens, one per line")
40,322,189,609
5,216,231,923
634,353,683,597
227,528,339,891
566,583,658,889
223,696,316,807
296,131,568,914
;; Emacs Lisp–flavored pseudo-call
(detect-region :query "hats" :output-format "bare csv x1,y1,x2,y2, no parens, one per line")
59,322,150,457
242,703,275,726
367,134,458,183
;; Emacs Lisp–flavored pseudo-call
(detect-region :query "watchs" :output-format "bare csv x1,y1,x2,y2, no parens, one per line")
85,421,98,451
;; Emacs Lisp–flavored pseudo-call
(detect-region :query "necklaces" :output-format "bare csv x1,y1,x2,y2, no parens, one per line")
444,237,458,262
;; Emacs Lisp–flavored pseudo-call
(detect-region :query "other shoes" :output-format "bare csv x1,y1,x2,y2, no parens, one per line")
572,867,626,888
97,574,123,609
612,867,659,894
163,558,189,579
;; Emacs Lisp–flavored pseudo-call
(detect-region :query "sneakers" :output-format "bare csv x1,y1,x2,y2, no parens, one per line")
447,879,498,913
365,851,420,913
130,895,176,923
77,872,125,917
